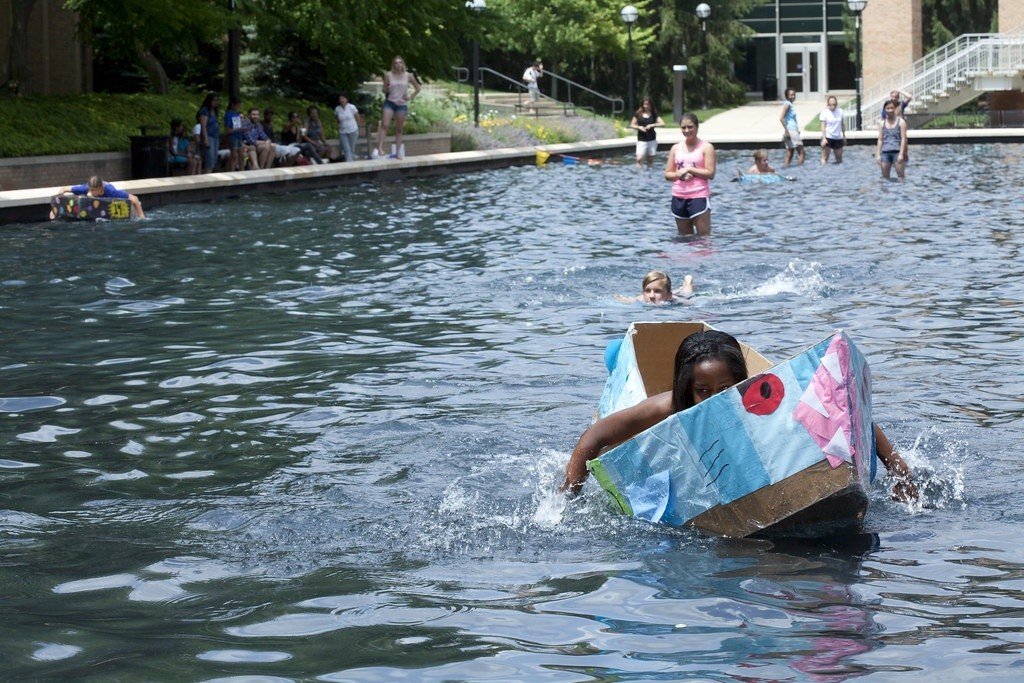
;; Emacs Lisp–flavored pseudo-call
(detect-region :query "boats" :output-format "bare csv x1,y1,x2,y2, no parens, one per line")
583,319,878,544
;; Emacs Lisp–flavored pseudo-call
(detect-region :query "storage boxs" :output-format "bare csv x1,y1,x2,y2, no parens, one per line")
590,321,774,455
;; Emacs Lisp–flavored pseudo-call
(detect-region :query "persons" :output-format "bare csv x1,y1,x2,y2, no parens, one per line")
876,101,907,177
522,61,544,115
820,95,849,165
167,93,327,176
58,175,145,220
629,95,666,170
664,113,717,235
378,55,421,160
747,149,776,173
558,331,918,497
642,271,696,304
881,88,912,162
779,88,804,166
332,91,361,162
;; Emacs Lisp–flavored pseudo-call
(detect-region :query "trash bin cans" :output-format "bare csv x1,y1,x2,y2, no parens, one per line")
762,76,777,100
127,134,169,179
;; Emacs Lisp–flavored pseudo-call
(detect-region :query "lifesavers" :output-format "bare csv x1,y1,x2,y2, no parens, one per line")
741,174,784,185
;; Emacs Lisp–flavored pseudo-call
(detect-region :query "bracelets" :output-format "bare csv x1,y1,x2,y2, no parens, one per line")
653,123,656,127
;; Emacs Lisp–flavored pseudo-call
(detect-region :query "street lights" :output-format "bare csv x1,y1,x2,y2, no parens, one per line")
464,0,487,128
620,5,640,122
846,0,868,131
695,2,712,110
